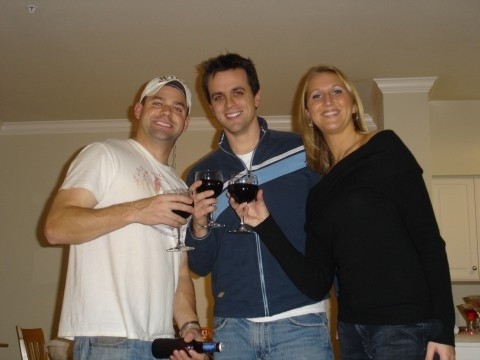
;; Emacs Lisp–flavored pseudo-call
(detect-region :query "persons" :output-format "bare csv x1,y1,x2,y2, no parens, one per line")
226,65,456,360
184,53,339,360
44,76,206,360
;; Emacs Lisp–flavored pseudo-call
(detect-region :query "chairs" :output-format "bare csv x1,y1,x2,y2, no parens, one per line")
16,324,45,360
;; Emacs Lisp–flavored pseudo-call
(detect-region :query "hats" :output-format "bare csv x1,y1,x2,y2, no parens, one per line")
140,75,192,115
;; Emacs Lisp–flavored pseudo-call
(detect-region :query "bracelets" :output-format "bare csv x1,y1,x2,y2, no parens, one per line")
179,321,201,339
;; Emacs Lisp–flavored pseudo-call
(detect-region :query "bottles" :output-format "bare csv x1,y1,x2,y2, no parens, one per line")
152,338,224,359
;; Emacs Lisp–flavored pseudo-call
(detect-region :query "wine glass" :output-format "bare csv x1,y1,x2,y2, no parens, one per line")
228,173,258,234
194,169,225,229
466,296,480,332
460,304,478,333
163,188,195,253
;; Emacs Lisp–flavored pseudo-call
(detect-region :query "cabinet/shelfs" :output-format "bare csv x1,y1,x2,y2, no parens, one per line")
433,175,480,281
